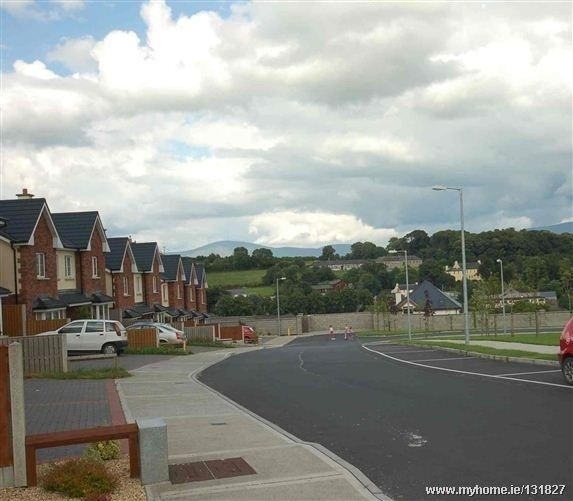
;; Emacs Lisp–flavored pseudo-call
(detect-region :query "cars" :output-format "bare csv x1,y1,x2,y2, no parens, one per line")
124,323,188,347
243,326,255,345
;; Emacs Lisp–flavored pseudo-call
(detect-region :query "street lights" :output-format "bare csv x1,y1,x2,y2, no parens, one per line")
432,185,471,346
496,259,506,337
276,277,286,336
387,249,412,346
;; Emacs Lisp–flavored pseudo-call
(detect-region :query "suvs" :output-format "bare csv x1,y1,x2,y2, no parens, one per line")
34,319,128,357
557,316,572,385
